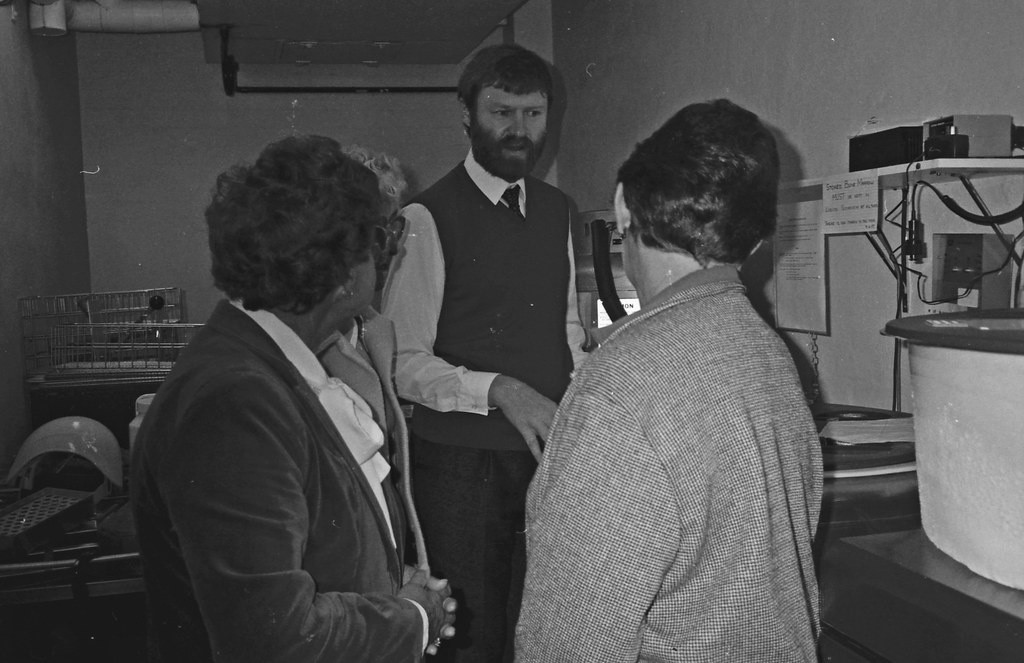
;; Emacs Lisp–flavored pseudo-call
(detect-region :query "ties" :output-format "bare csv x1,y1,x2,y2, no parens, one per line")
501,183,525,225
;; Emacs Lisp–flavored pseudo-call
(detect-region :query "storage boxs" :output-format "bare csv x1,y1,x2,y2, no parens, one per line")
880,306,1024,595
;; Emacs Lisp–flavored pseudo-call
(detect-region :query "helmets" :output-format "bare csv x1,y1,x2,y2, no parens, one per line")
3,416,123,505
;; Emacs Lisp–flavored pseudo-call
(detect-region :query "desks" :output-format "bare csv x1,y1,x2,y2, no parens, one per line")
0,495,147,663
806,399,920,540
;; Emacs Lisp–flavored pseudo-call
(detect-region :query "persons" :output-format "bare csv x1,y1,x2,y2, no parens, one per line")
512,97,827,663
380,42,593,663
134,134,461,663
309,142,434,587
